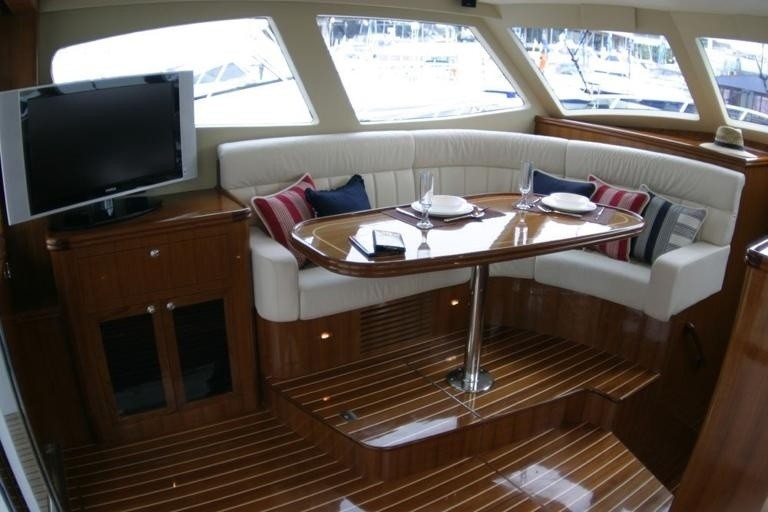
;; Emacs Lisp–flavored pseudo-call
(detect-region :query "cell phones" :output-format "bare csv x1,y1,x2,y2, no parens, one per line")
349,231,376,258
373,228,406,255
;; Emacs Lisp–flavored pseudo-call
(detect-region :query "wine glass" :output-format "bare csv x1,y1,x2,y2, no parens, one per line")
416,172,433,228
515,159,535,210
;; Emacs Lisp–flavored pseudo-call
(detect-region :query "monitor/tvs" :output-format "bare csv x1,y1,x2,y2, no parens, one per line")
0,68,199,233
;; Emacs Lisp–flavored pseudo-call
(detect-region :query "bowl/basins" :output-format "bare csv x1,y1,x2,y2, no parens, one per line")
428,196,464,211
550,193,589,206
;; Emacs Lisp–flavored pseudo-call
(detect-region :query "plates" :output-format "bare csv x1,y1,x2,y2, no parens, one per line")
542,191,597,212
411,195,473,217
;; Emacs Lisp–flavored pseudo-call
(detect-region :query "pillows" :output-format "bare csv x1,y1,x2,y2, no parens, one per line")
305,174,371,216
629,185,707,265
586,174,651,263
533,169,597,200
251,173,317,267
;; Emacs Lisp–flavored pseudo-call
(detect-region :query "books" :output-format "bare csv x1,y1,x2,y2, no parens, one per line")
349,233,381,257
372,229,406,252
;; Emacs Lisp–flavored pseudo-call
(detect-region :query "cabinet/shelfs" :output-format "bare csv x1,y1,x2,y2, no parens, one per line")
43,185,260,445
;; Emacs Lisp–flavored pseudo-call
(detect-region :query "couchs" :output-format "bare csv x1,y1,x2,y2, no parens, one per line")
217,128,746,324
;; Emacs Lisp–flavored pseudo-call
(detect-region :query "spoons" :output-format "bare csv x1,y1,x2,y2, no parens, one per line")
444,211,485,223
537,205,582,218
